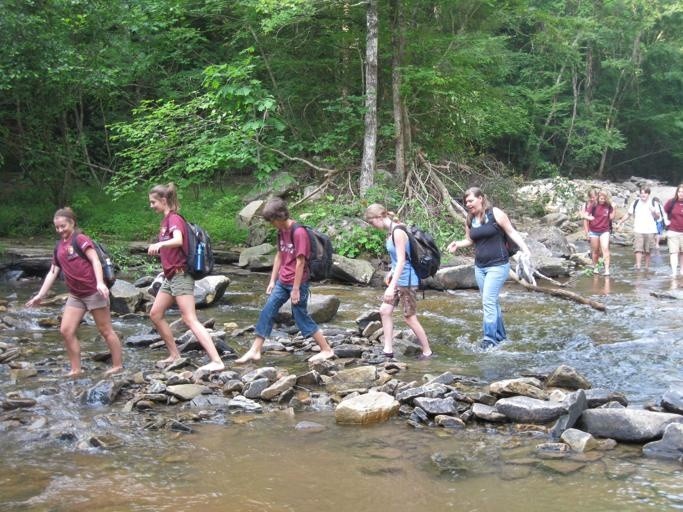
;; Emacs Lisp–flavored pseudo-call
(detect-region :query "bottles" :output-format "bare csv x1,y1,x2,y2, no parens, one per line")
106,258,116,283
194,244,204,272
420,255,432,278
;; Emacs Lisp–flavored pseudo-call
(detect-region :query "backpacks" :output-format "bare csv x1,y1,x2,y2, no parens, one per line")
392,224,441,279
167,212,214,279
55,234,116,289
291,223,333,282
487,208,519,256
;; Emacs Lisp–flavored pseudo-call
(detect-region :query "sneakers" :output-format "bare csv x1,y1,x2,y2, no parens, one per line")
480,342,494,349
148,272,165,297
379,351,394,358
414,352,434,361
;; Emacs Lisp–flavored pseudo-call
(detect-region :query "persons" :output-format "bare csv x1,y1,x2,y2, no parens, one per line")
147,182,224,372
234,197,334,364
26,207,123,377
364,204,434,358
581,184,683,277
446,187,531,351
